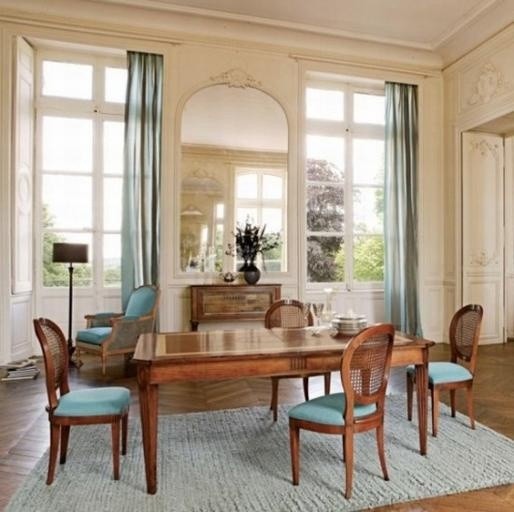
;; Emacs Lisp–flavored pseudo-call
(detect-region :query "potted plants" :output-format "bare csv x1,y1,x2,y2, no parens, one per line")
224,214,282,284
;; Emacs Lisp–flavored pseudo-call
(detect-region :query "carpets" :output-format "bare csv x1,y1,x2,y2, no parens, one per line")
4,393,514,511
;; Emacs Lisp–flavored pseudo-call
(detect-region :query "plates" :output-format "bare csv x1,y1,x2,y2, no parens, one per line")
331,313,368,336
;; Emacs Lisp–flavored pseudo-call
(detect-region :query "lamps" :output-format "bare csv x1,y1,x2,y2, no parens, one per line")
53,241,90,366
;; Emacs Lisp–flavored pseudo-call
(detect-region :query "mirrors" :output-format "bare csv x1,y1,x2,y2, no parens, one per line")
167,68,297,289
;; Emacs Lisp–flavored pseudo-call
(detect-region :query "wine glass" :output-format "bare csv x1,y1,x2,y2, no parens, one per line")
312,303,323,337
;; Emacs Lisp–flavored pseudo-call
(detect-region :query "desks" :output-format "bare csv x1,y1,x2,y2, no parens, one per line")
188,283,283,332
129,326,436,496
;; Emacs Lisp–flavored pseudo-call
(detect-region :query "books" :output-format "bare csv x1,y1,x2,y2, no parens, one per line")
0,356,41,382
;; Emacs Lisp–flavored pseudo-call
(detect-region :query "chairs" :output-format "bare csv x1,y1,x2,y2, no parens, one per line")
33,318,132,487
75,283,163,377
406,303,485,437
265,299,397,499
